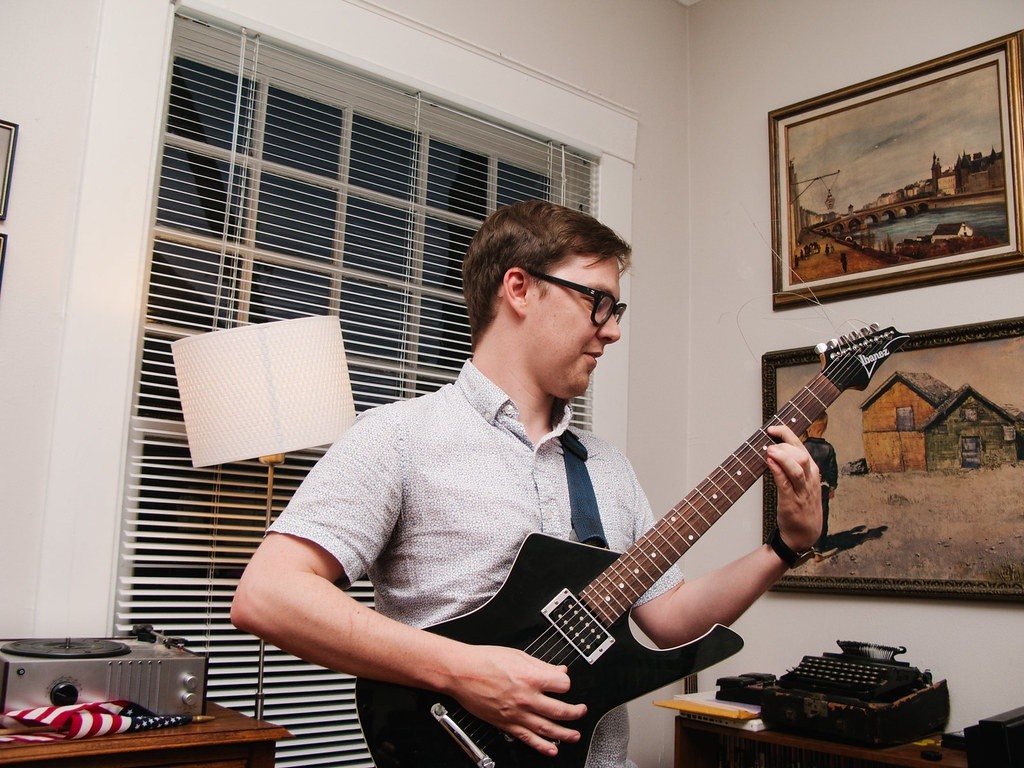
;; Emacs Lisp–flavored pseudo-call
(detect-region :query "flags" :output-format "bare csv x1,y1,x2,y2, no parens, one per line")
0,699,193,744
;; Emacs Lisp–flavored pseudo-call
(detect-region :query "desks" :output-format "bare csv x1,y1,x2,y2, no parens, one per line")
673,714,967,768
0,701,295,768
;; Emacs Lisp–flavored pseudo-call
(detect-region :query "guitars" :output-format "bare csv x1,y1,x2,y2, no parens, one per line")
356,322,911,768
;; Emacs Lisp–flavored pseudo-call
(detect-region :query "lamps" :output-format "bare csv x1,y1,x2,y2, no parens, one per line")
167,314,358,726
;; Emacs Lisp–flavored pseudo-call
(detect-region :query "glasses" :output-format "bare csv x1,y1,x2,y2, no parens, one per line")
525,270,627,326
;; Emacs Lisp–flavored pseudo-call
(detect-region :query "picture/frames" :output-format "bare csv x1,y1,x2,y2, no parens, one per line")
766,25,1024,317
762,316,1024,606
0,232,9,296
0,120,20,220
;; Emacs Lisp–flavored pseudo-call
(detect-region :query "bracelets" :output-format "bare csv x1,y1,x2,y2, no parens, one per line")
764,525,813,569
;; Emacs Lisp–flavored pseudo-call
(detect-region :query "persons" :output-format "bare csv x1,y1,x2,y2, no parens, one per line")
230,198,827,767
